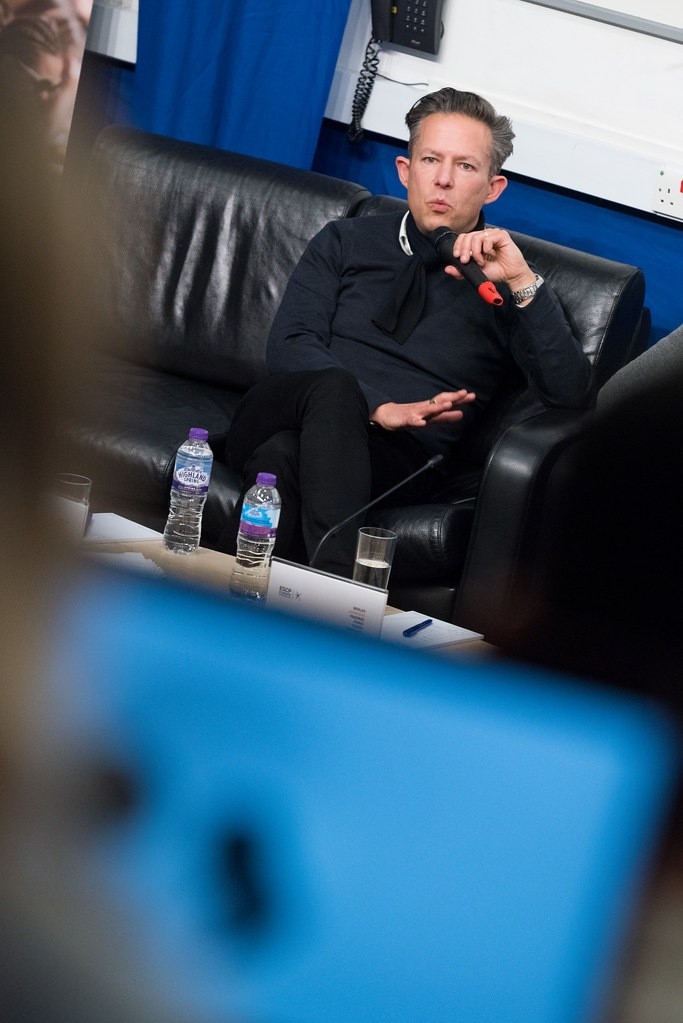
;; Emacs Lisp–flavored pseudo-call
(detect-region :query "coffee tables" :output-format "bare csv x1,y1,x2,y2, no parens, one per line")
59,537,489,675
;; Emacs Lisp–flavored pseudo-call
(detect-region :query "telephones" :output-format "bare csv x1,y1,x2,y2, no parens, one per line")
371,0,443,55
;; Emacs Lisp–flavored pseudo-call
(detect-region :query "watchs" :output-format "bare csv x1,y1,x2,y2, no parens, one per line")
500,271,548,304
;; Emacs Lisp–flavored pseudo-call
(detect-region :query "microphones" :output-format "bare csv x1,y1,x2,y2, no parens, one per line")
310,456,440,567
431,225,504,306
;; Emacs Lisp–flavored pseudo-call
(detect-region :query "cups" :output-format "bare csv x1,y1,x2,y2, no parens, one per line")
55,473,93,506
353,525,398,591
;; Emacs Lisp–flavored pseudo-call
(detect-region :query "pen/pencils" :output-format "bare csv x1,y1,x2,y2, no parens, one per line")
403,619,433,637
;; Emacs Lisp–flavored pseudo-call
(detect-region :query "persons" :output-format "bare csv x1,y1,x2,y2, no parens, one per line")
2,15,67,115
236,84,599,582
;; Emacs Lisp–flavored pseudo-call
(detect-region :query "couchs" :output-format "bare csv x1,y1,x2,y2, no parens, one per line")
34,125,651,645
14,558,672,1023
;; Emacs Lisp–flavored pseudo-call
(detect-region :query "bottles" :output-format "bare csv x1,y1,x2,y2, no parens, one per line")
161,427,213,556
230,471,282,606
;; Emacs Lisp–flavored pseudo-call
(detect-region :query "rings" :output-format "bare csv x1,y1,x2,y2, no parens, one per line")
481,228,489,241
429,396,438,404
